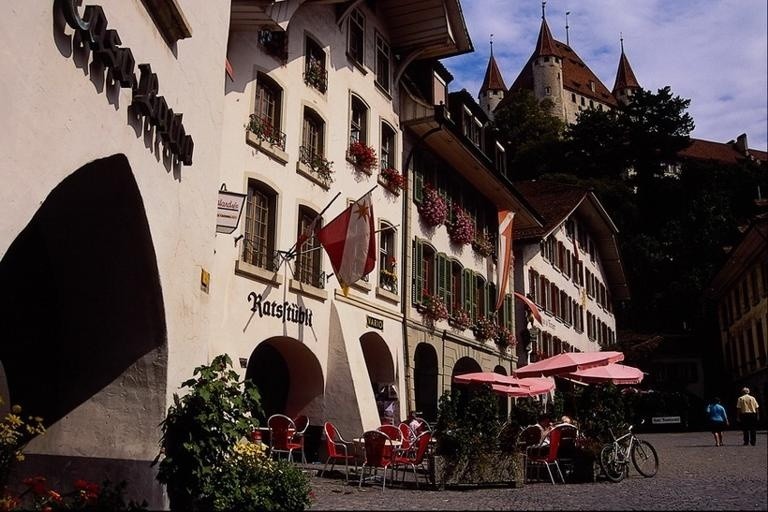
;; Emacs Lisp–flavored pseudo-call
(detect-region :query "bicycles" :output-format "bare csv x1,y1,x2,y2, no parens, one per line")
599,418,660,482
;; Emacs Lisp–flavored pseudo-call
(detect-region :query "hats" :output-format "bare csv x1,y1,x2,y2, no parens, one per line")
743,387,750,393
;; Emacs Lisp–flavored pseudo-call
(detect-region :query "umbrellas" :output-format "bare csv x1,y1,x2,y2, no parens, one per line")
490,376,555,397
513,349,625,379
454,371,521,387
564,363,644,385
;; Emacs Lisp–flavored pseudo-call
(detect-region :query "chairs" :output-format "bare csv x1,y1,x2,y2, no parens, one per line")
357,429,400,492
526,423,579,484
320,421,363,482
392,427,431,484
250,412,580,468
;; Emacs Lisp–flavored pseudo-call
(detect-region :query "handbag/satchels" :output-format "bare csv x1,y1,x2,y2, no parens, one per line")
704,412,710,416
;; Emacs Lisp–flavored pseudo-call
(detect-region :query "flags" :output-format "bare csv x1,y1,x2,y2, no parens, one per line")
314,192,377,296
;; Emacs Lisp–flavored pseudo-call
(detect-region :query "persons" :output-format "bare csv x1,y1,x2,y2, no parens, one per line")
736,386,760,446
407,415,420,432
533,412,553,447
706,398,729,446
